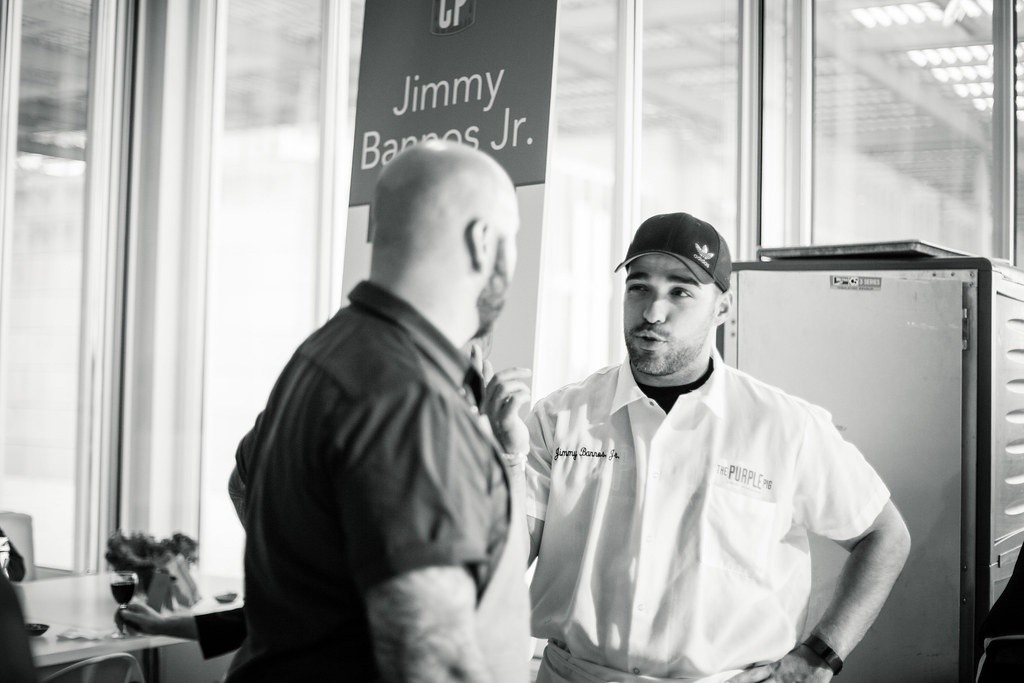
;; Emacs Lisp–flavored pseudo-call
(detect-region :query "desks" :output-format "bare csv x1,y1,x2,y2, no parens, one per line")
11,567,246,669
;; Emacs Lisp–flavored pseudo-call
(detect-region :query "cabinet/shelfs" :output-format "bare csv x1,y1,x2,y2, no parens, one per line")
716,253,1024,683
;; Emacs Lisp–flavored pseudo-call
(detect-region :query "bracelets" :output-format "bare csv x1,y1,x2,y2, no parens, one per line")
803,635,844,676
503,451,530,468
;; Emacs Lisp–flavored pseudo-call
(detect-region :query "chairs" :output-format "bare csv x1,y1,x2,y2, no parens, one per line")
40,653,147,683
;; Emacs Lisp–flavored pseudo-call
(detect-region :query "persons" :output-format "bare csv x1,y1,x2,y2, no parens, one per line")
471,213,912,683
115,139,533,683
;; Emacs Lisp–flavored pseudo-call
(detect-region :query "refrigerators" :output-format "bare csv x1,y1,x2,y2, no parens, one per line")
717,257,1023,683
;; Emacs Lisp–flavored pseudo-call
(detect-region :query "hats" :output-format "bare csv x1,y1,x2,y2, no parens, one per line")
614,212,733,292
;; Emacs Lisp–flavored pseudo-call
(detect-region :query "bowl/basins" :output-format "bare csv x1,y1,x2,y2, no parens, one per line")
24,624,49,637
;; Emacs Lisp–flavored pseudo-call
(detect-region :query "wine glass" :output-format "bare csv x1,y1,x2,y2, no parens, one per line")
109,572,137,638
